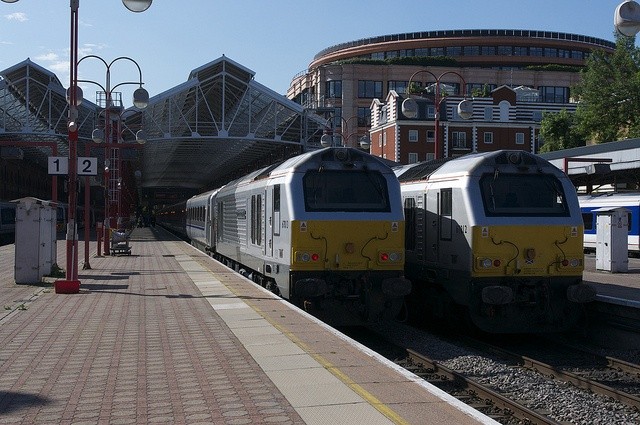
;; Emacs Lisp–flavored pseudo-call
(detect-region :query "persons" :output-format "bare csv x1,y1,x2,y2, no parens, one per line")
137,214,144,228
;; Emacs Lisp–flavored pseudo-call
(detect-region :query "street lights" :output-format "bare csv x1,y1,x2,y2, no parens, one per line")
403,69,473,159
65,56,150,271
92,109,146,255
3,0,153,294
320,115,370,153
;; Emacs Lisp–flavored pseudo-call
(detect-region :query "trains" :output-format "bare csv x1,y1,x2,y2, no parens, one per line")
527,133,635,263
152,146,410,328
387,150,596,340
